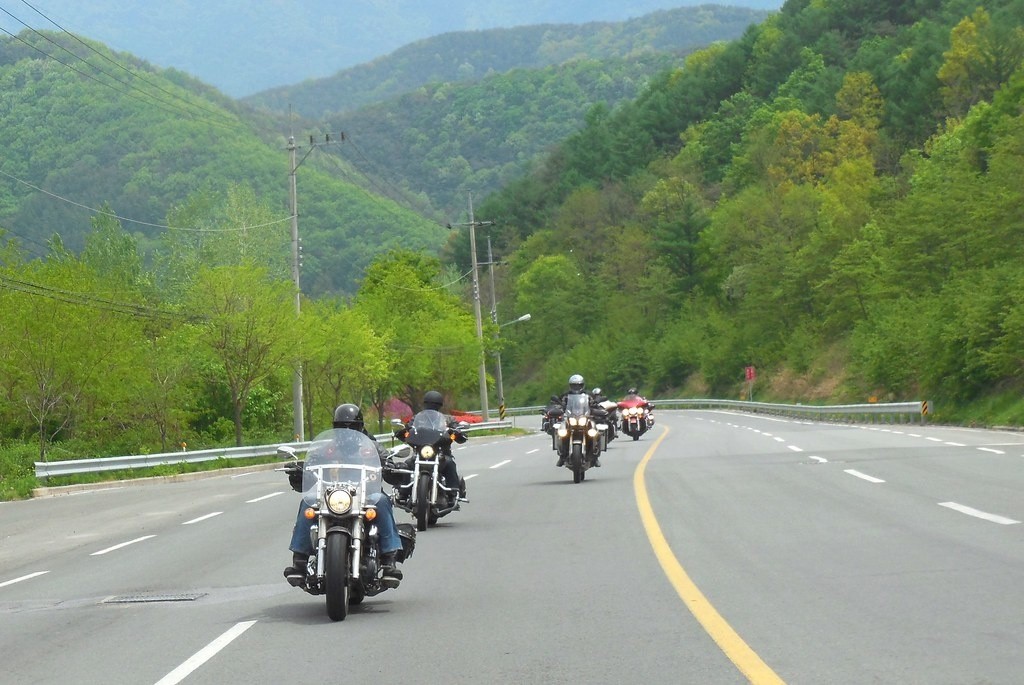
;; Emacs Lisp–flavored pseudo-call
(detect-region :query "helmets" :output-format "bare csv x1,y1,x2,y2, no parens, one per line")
424,391,443,410
592,388,601,397
628,388,637,394
569,374,585,394
333,403,364,432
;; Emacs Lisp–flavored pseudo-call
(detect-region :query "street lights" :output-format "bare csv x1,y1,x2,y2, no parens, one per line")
489,311,531,423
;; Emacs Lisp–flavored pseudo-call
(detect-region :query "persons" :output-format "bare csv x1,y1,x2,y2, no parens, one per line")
283,404,404,581
592,388,618,438
621,389,655,428
548,375,607,468
540,395,565,432
395,390,468,502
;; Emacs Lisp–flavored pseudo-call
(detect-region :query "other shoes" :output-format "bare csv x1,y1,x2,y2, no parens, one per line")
614,432,618,438
557,454,566,467
448,496,459,508
397,493,407,504
594,460,601,467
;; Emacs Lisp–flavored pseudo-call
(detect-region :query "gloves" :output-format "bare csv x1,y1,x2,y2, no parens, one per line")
449,428,458,436
285,462,297,474
384,461,395,476
395,428,404,439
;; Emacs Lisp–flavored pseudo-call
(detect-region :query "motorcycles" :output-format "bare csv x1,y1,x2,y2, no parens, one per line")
388,418,471,533
538,400,566,436
266,426,416,624
540,393,609,483
595,396,621,443
616,394,655,441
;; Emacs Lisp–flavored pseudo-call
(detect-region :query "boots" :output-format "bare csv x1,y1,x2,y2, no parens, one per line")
284,553,308,580
381,551,402,580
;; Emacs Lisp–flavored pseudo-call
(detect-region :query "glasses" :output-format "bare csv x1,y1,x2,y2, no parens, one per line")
340,423,356,429
